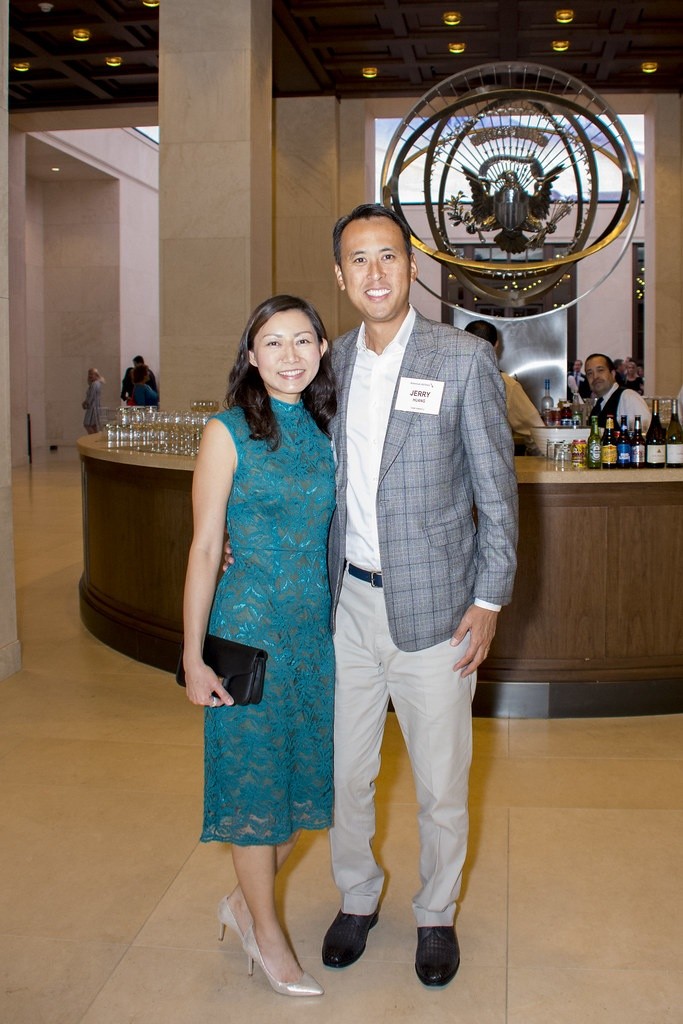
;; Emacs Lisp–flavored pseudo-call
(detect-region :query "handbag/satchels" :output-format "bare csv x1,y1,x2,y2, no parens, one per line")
175,630,268,706
126,387,138,407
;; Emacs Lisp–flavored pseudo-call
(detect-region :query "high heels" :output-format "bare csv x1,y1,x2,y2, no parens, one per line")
242,923,325,997
218,894,244,941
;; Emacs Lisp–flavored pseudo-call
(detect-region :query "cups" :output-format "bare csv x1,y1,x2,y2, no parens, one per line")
547,439,565,460
554,444,570,460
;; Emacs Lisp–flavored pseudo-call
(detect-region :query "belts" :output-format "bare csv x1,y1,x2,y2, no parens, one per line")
343,558,383,588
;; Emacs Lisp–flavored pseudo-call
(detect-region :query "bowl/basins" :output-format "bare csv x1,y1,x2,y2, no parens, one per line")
530,427,605,460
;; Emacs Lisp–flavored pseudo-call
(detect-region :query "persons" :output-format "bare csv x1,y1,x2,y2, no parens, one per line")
121,356,159,412
81,368,106,434
568,354,652,437
461,319,548,458
220,204,518,988
182,294,336,997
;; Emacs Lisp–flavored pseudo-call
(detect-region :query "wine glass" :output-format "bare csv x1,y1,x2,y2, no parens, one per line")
103,400,219,456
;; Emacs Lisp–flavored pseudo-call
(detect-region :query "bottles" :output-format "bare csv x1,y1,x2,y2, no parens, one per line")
588,416,602,469
602,415,617,468
543,380,582,425
646,399,666,468
631,415,646,468
580,440,588,464
571,440,583,464
666,399,683,468
617,415,631,468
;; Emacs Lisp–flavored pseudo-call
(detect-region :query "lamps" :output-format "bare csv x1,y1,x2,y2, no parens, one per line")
444,11,462,25
144,0,160,7
363,68,376,78
73,28,92,41
642,60,659,74
12,62,30,71
107,55,122,66
553,42,569,52
556,8,575,25
450,42,466,53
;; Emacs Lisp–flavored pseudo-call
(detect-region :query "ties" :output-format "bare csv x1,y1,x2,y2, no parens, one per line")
591,397,604,415
574,373,578,384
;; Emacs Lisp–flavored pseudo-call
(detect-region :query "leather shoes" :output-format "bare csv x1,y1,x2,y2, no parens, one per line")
415,926,460,987
321,902,378,968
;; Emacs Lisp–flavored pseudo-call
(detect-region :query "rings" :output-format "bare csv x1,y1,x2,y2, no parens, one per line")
211,698,218,708
